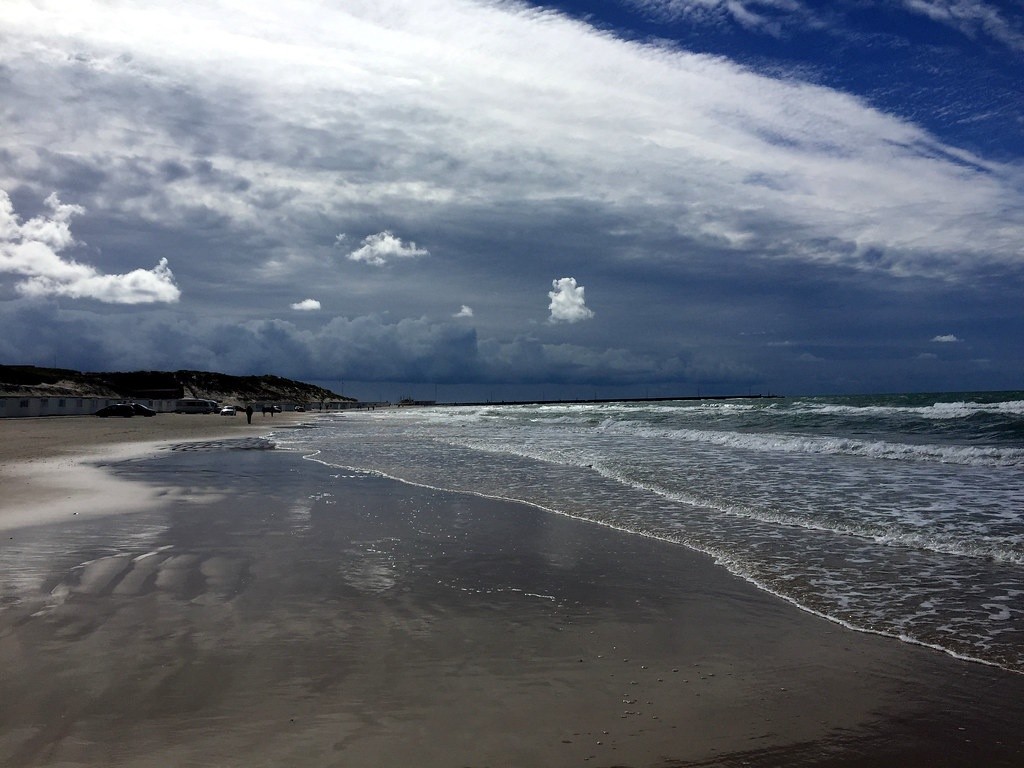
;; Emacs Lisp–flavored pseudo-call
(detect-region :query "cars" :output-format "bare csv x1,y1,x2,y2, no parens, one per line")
94,404,133,418
220,406,236,416
126,403,156,417
297,407,306,412
294,406,300,411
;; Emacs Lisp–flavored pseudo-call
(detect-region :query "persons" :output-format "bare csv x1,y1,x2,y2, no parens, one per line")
270,405,275,417
261,406,266,417
245,406,254,424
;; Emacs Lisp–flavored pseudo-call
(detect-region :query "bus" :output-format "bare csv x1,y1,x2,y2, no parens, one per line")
175,398,213,415
